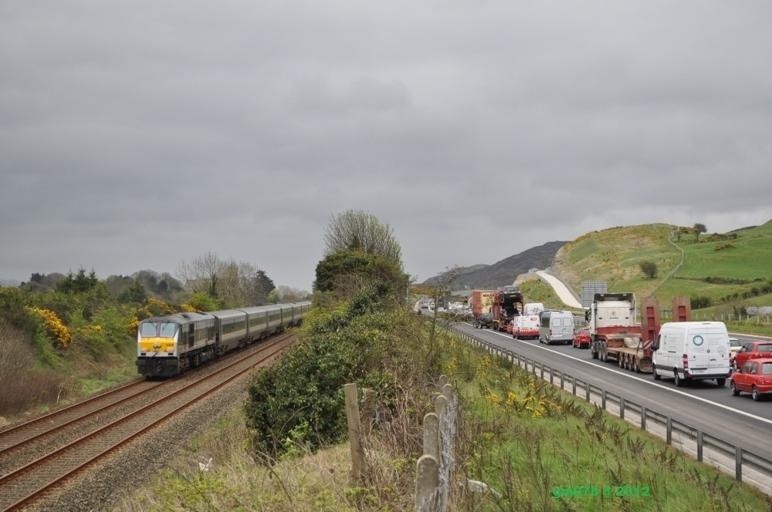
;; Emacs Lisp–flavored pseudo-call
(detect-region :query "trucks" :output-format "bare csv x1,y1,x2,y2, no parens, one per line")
411,286,771,400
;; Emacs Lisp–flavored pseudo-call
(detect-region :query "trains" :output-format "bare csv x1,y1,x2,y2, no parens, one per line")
135,301,315,381
